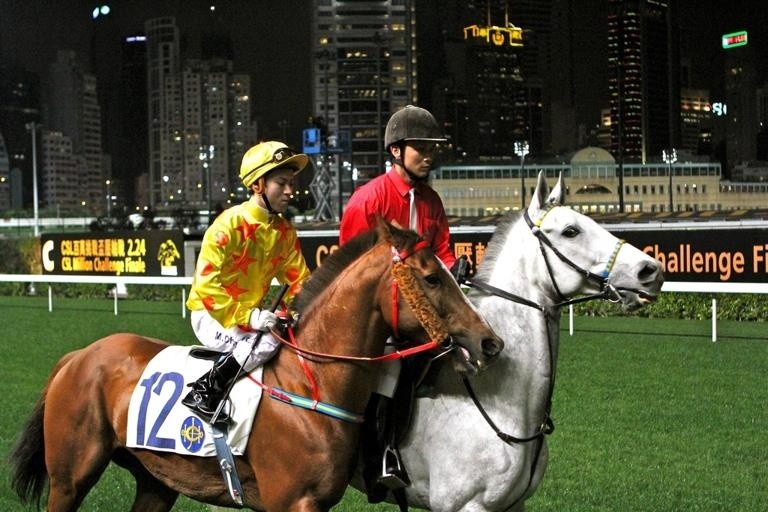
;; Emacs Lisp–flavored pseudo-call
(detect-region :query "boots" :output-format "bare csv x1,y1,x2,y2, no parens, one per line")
182,352,247,420
364,392,402,503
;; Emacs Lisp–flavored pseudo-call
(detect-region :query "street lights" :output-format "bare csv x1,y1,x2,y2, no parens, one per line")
514,141,531,209
663,147,677,211
199,145,215,228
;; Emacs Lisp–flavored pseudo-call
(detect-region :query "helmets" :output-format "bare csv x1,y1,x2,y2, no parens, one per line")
238,141,309,188
384,105,448,153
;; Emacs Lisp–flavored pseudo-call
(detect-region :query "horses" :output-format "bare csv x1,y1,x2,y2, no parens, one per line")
9,215,506,512
348,167,666,512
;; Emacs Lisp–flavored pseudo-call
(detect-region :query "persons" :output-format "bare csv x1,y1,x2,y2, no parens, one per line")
180,139,314,432
338,103,474,505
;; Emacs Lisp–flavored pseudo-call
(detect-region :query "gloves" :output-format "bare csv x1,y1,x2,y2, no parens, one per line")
451,255,469,285
249,308,278,332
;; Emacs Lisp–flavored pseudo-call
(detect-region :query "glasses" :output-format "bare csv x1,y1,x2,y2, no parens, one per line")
242,148,295,181
